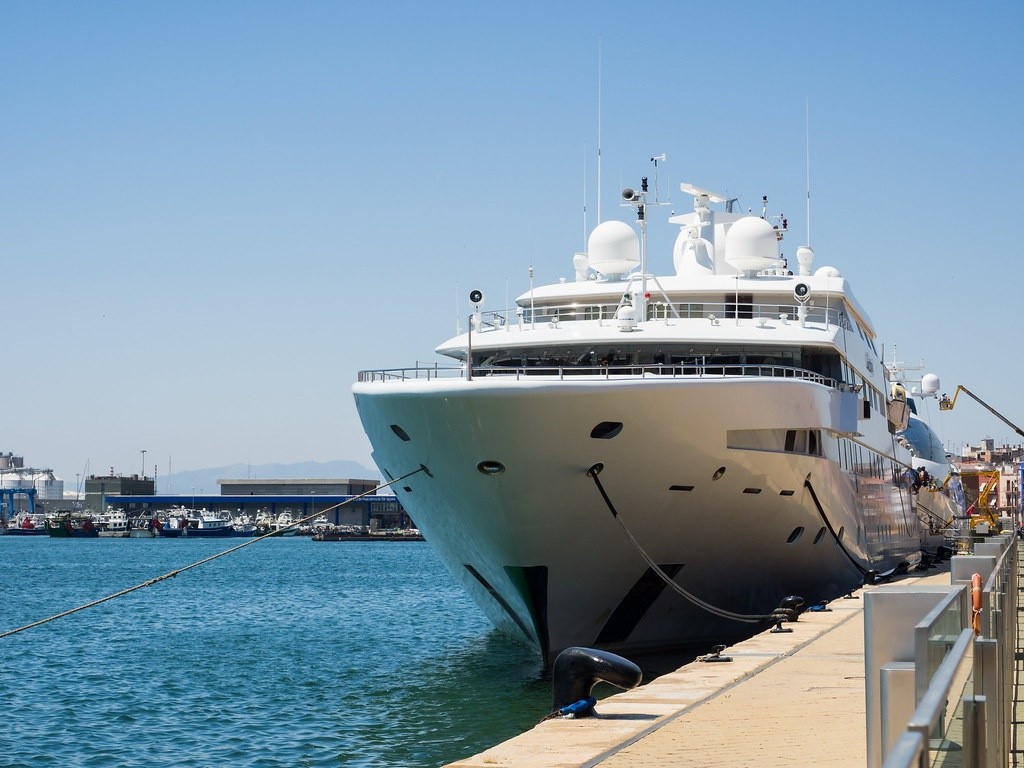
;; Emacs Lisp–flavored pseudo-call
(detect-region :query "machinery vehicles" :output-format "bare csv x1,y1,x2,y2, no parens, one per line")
926,469,1003,537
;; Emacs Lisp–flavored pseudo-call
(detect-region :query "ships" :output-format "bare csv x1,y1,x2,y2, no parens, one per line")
352,44,960,677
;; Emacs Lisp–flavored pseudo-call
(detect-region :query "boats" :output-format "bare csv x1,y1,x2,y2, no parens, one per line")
4,506,333,539
